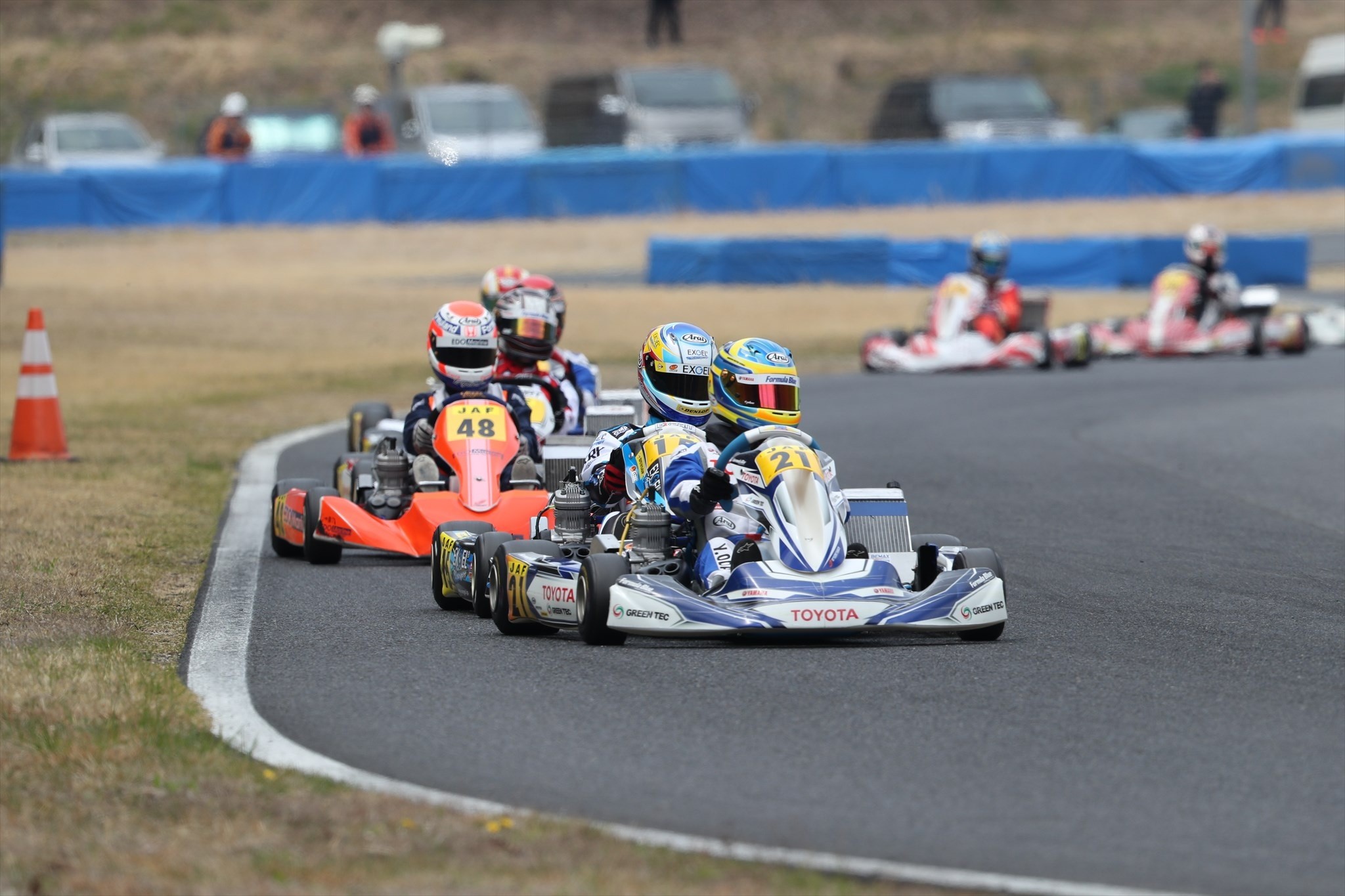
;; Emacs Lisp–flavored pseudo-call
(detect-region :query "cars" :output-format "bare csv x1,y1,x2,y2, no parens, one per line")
7,112,169,170
380,83,542,160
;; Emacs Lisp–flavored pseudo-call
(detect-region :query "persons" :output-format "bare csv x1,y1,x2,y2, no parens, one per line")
641,0,684,50
661,336,869,597
341,79,389,161
202,89,252,163
1248,0,1294,40
1182,222,1240,331
477,266,601,438
579,316,716,541
1184,55,1233,141
406,299,538,496
965,228,1025,343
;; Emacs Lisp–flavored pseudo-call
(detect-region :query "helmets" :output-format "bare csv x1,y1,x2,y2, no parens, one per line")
707,337,802,430
515,277,565,341
427,301,500,387
1184,224,1228,271
493,287,557,360
480,264,528,310
637,322,718,426
969,231,1013,279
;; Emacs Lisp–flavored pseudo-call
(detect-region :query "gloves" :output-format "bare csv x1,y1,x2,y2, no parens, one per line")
692,467,734,504
413,419,434,449
512,436,528,460
604,458,626,493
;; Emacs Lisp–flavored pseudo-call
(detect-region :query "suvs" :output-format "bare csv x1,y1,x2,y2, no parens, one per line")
868,74,1086,145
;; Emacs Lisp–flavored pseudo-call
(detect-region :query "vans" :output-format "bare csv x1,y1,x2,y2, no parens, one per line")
544,64,748,147
1287,31,1345,133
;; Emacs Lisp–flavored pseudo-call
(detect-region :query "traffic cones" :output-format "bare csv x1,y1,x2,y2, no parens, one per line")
0,307,84,467
